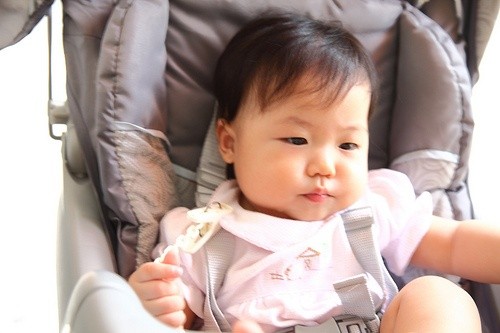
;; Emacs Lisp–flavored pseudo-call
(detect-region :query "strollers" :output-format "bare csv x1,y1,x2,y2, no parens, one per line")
1,0,500,333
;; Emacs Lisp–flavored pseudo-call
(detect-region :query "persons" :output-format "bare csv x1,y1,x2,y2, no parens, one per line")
125,6,500,333
